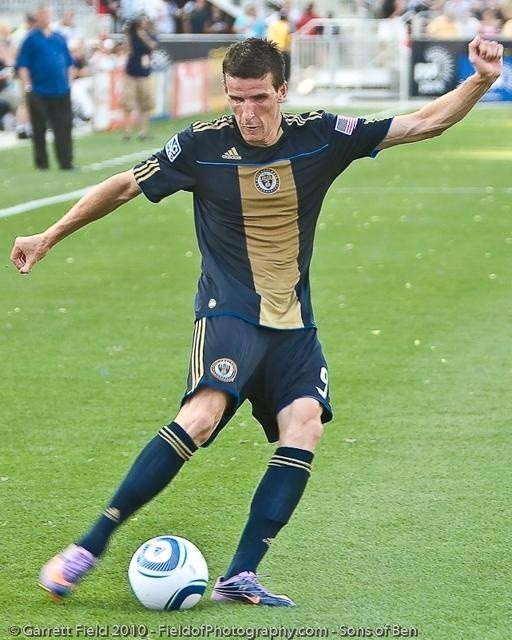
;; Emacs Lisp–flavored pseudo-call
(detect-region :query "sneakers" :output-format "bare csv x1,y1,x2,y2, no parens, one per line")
212,572,296,607
39,543,97,597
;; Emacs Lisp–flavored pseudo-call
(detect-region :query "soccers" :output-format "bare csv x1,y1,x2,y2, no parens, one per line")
128,536,209,613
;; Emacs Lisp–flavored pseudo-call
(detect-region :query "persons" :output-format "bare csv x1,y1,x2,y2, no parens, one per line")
1,0,512,171
10,35,505,609
